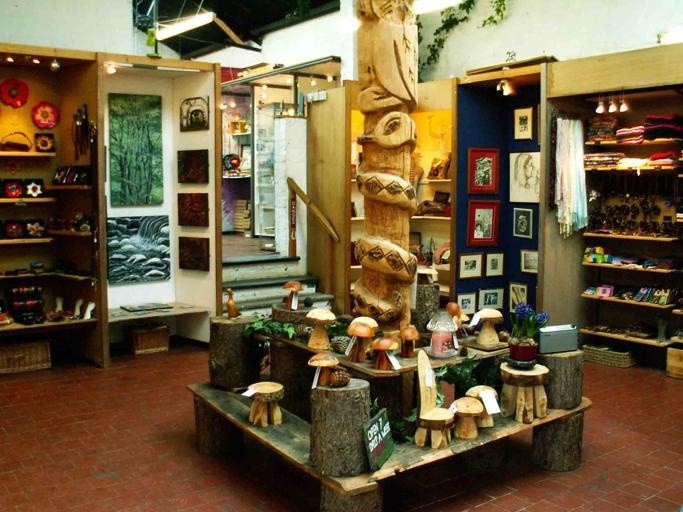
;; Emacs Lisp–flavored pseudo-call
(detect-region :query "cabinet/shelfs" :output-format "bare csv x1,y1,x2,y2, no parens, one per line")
343,78,458,297
186,303,592,512
222,56,341,237
0,43,223,373
546,42,683,379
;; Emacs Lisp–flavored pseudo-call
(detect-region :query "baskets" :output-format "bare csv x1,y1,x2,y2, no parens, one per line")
0,342,52,373
582,344,637,368
130,323,169,354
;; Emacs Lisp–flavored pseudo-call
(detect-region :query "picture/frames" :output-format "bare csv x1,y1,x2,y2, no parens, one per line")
509,282,528,313
478,287,505,311
457,146,541,281
512,106,534,142
457,292,477,315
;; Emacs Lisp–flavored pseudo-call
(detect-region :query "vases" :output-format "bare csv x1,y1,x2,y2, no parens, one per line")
507,344,538,370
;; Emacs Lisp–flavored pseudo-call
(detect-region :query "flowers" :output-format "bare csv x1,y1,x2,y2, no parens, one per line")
508,303,550,346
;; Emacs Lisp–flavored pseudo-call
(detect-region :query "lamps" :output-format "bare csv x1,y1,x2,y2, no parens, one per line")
496,79,513,99
